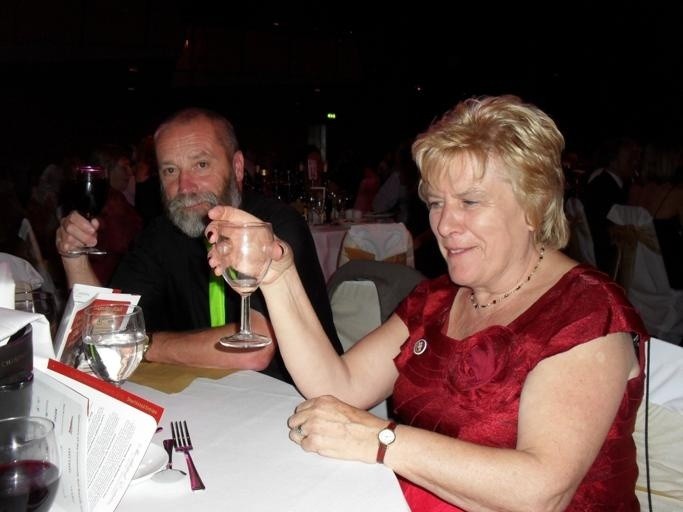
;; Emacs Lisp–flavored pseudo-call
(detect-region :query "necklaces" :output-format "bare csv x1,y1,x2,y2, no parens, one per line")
469,244,546,309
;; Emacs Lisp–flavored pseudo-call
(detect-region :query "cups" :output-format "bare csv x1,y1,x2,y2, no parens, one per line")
13,291,57,342
1,324,34,468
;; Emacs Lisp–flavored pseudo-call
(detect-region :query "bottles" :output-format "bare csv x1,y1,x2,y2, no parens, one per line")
323,200,328,224
308,194,315,207
242,163,286,202
302,206,309,227
316,197,324,225
309,204,322,226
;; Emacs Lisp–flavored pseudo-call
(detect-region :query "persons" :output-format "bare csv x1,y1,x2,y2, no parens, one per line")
584,122,642,228
54,108,345,387
353,139,402,216
629,139,683,291
204,96,650,512
560,132,623,267
94,139,143,254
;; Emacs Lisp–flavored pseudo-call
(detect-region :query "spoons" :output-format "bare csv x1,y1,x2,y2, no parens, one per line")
151,439,186,485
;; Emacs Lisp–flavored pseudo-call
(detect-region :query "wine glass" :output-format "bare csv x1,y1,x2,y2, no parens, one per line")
328,191,342,225
65,167,109,255
0,417,63,511
83,304,148,388
14,283,35,314
218,221,273,350
342,193,351,222
333,197,346,223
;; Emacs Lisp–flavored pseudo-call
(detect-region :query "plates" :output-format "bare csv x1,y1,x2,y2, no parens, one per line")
132,443,169,483
365,212,393,219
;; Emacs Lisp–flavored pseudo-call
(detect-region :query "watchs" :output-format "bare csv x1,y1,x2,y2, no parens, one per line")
376,419,400,464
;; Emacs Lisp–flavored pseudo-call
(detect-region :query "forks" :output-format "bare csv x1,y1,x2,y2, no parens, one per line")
170,421,204,491
